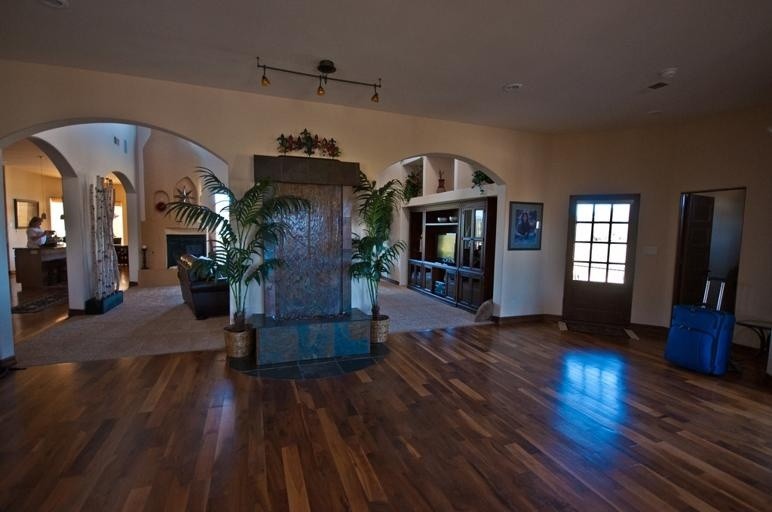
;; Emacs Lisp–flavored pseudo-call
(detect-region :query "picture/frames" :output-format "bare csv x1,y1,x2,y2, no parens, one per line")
507,201,544,250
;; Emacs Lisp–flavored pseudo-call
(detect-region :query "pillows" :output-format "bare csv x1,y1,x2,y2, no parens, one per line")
475,300,494,322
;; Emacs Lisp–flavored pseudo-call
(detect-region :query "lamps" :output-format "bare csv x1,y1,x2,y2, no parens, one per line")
258,60,384,104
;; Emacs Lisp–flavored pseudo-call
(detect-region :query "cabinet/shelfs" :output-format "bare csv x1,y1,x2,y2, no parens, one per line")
407,260,483,315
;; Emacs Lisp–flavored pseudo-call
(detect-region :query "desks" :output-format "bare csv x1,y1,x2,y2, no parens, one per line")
736,320,772,370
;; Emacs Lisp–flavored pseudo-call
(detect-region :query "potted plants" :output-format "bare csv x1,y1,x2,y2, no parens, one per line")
166,165,311,371
345,170,409,342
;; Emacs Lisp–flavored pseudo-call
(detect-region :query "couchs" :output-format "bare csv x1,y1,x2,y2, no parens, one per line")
174,254,230,320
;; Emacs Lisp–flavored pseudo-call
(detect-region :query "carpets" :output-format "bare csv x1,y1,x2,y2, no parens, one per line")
12,266,495,367
558,321,639,341
12,290,68,315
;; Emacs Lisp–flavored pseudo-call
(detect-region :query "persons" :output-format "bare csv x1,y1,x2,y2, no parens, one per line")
515,212,531,240
25,216,51,248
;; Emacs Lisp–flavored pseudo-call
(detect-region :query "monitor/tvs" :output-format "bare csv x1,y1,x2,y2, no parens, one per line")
436,233,457,264
